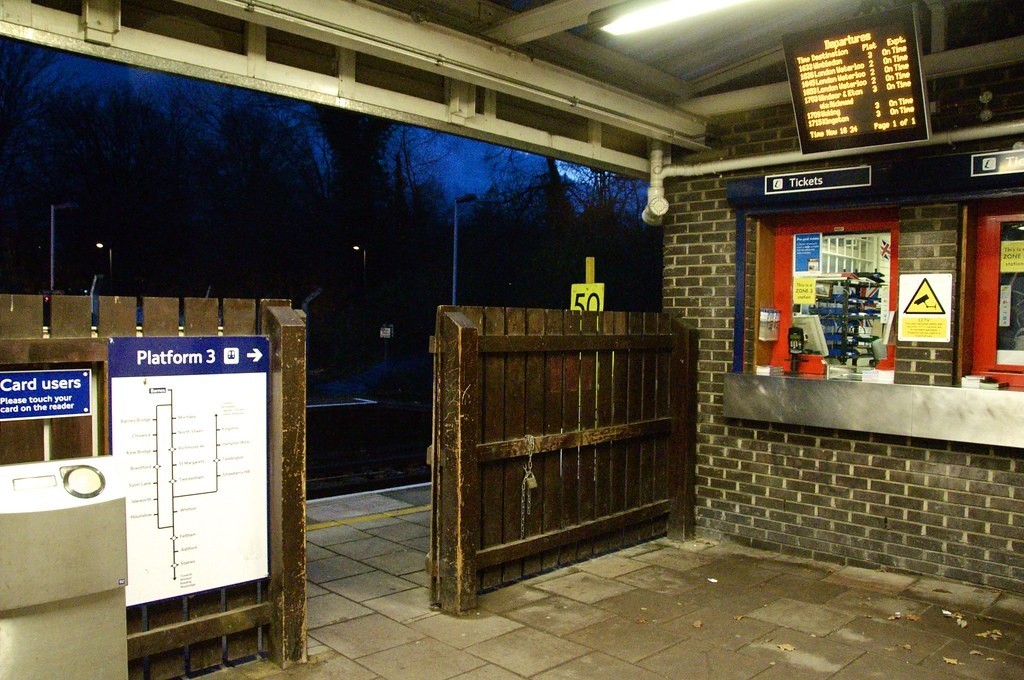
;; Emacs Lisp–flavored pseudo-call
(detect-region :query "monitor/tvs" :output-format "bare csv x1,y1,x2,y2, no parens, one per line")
793,315,829,357
781,2,932,156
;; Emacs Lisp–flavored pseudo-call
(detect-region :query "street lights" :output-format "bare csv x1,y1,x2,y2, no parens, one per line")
451,193,476,305
90,238,117,289
349,243,369,349
47,198,70,289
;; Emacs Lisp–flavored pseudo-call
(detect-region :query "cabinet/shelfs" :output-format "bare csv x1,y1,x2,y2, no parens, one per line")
802,278,885,369
